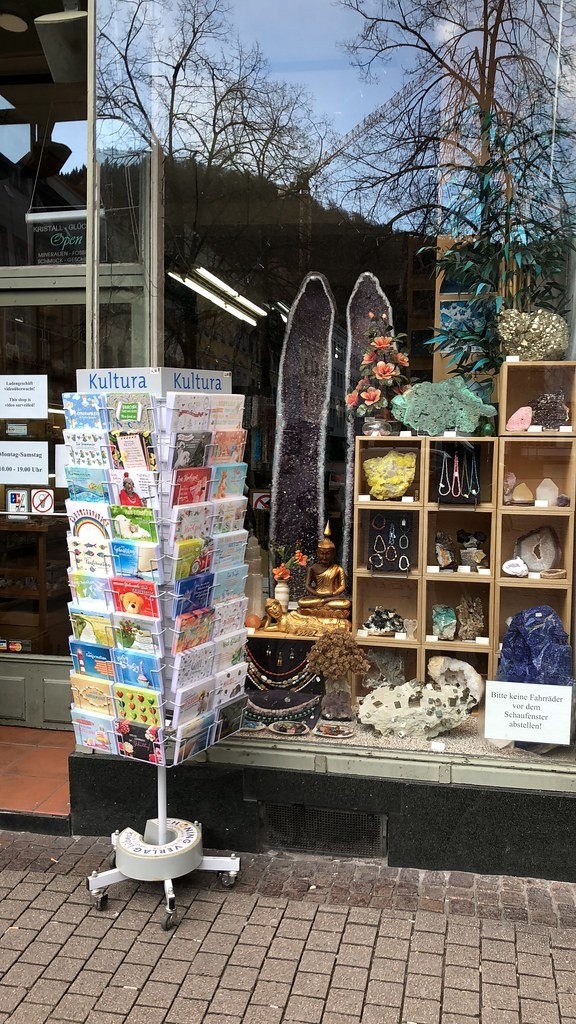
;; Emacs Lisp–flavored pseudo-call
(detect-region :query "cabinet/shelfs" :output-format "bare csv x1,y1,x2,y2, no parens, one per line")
347,360,576,720
0,412,73,656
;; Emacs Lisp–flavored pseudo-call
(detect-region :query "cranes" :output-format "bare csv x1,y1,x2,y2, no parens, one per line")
277,64,444,284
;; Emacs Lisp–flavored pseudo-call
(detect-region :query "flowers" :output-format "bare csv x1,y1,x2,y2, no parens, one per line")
345,310,421,416
268,539,308,582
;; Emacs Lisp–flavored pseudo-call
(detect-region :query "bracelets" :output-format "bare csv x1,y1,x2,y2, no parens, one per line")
385,545,397,561
374,535,386,553
399,534,408,549
399,555,410,571
388,523,396,545
372,513,385,530
243,642,321,722
400,513,411,534
369,553,383,568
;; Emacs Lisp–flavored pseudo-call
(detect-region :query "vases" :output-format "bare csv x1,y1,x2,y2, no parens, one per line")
275,580,290,613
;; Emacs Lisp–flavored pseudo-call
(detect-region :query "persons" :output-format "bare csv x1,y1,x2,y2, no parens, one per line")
298,520,350,608
263,597,352,637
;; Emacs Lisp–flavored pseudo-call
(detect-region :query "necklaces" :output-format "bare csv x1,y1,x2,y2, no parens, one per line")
460,455,470,498
438,454,451,496
470,458,480,496
451,454,461,498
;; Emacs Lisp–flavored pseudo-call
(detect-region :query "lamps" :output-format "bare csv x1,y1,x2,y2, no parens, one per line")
166,264,268,326
